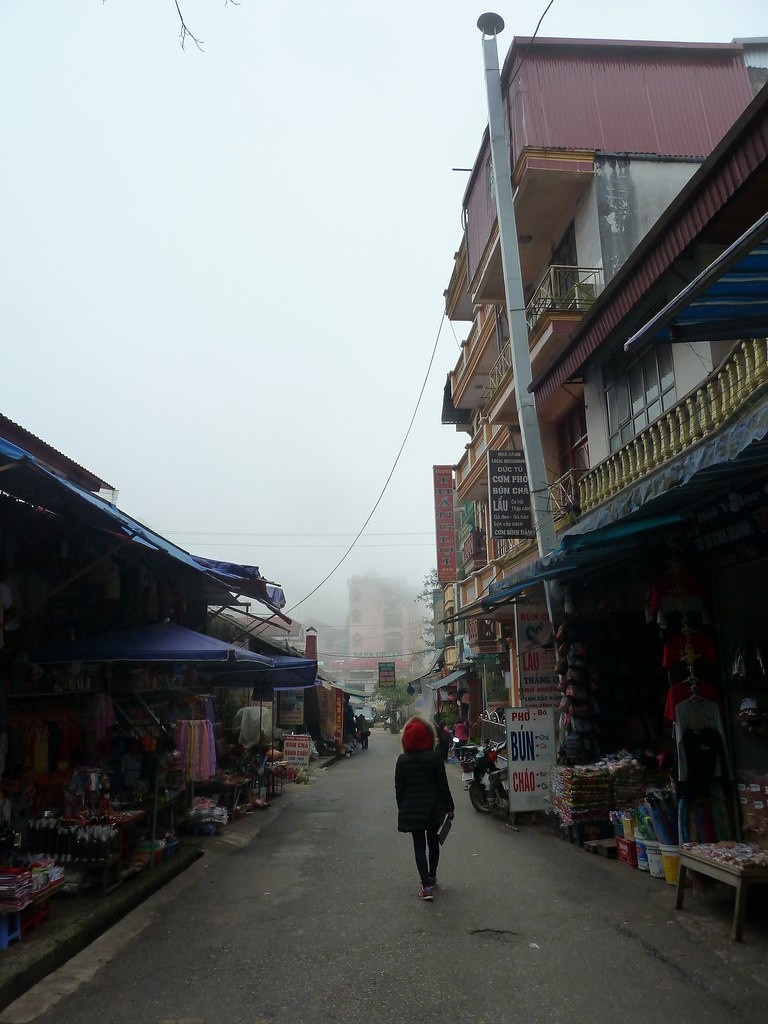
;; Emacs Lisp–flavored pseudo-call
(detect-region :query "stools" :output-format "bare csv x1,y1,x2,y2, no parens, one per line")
0,890,49,950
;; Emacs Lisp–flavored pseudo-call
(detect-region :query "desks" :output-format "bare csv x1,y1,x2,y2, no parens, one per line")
675,841,768,944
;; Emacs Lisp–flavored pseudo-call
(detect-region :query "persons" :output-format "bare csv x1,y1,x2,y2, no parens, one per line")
0,788,13,846
356,714,369,751
433,718,470,764
393,714,457,901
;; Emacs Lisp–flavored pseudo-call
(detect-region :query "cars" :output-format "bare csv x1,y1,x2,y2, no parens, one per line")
353,706,375,728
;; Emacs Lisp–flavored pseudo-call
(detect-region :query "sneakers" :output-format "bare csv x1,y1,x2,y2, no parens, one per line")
417,885,435,900
428,875,437,887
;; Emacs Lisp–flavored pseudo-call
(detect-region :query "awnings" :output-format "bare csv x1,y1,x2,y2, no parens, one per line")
478,395,768,613
196,649,319,693
17,613,279,676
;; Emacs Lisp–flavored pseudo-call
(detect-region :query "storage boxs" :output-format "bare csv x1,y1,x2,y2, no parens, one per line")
615,837,635,865
634,833,683,885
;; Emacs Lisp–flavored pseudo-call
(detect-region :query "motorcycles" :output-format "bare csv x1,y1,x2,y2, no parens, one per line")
461,738,515,818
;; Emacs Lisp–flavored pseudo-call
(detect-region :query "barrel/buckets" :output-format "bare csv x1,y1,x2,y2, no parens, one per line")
635,830,652,871
644,838,667,878
659,841,691,885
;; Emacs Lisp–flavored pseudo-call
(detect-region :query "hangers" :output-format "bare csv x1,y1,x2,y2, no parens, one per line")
177,703,211,725
677,607,701,699
